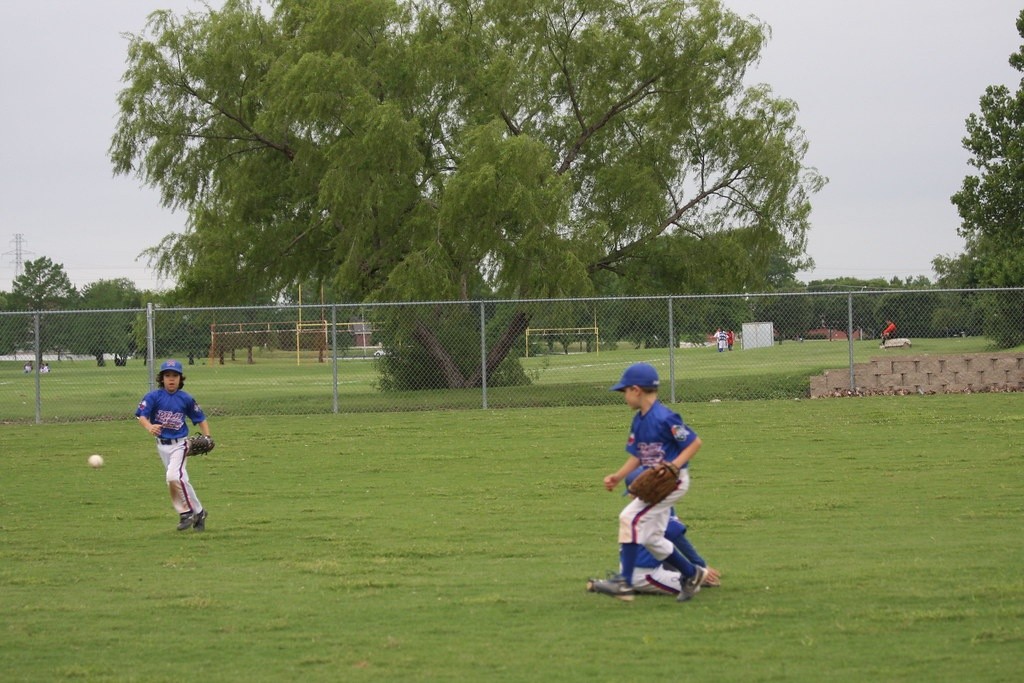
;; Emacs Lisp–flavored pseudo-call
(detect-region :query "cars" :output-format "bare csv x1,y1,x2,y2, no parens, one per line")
373,348,395,358
645,329,681,349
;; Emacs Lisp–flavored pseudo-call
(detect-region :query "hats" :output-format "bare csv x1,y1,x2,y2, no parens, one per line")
624,466,646,496
160,361,183,374
609,362,660,391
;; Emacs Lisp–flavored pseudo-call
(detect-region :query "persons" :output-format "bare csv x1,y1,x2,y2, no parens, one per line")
586,362,721,603
23,360,50,373
714,327,735,353
879,318,897,350
135,360,210,532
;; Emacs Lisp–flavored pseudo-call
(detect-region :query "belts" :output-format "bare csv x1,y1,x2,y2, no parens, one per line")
155,437,177,444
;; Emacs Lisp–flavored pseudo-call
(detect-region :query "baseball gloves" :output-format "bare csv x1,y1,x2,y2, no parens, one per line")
184,432,215,457
626,459,682,505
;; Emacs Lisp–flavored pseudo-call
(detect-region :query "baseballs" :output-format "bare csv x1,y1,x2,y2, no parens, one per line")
88,454,104,470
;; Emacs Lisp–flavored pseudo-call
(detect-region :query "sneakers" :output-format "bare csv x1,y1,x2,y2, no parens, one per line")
193,509,206,531
677,564,708,602
177,511,194,531
586,570,635,602
